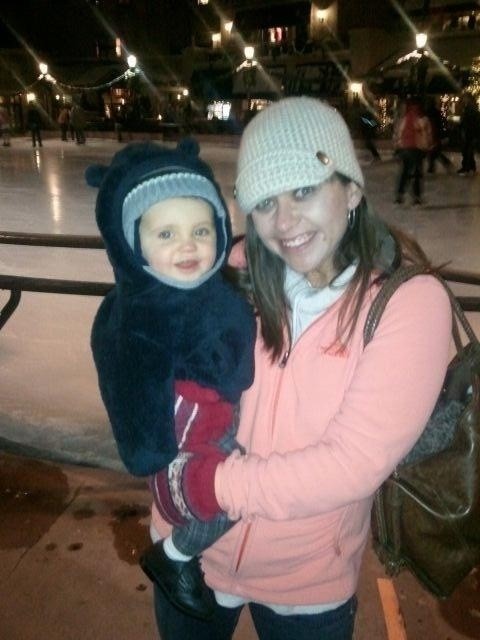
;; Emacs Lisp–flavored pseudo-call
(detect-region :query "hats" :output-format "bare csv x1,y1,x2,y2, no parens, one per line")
233,96,365,215
121,166,226,251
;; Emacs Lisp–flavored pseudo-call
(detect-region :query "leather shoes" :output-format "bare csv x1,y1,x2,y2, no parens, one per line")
139,539,217,620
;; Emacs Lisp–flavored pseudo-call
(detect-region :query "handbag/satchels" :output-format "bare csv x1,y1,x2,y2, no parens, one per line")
365,266,480,598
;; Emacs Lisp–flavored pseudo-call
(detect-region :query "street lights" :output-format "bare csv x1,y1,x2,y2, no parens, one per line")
414,30,430,116
37,62,52,135
240,44,256,116
126,54,141,133
351,81,362,104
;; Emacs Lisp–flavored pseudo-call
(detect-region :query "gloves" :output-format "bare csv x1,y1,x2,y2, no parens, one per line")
148,380,233,526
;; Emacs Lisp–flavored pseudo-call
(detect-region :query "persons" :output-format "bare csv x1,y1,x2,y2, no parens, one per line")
151,87,454,639
456,95,478,176
82,132,258,619
394,94,432,207
1,94,455,179
